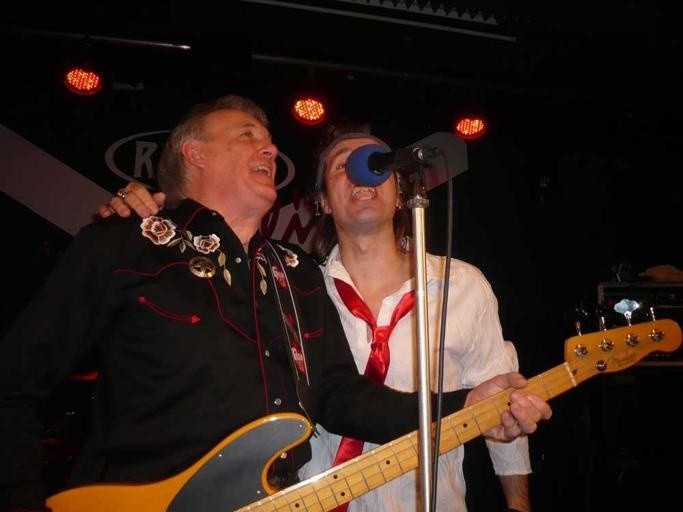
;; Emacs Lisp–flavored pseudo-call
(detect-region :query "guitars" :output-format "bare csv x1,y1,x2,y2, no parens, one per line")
47,299,682,511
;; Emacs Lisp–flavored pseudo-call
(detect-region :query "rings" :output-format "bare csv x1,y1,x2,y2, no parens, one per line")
117,189,131,203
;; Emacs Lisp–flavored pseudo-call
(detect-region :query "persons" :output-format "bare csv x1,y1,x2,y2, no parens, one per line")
1,94,552,512
98,130,533,512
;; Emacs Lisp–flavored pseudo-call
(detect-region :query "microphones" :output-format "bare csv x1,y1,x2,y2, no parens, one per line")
345,143,441,187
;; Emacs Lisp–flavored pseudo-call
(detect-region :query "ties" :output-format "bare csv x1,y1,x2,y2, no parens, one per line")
324,277,416,512
